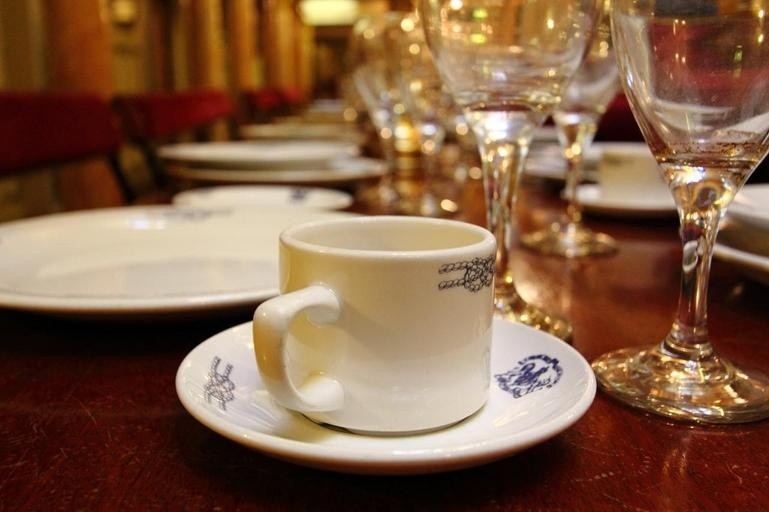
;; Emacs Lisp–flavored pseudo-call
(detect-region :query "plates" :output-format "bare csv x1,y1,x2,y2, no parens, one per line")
174,314,600,470
0,104,386,310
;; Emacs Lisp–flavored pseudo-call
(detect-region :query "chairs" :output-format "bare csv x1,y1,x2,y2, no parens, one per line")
0,75,345,219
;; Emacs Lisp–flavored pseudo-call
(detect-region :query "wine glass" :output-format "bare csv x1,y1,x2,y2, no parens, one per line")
351,2,769,430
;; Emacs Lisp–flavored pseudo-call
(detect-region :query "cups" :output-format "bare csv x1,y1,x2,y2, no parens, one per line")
251,213,496,436
